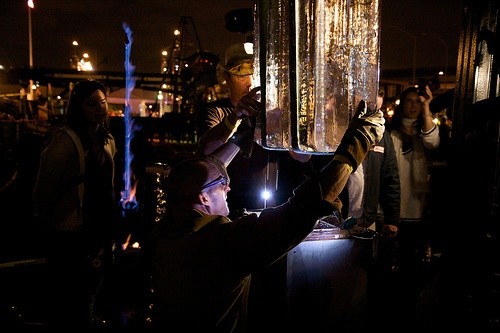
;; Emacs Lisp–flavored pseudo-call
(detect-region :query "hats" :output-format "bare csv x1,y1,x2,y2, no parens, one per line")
222,43,256,76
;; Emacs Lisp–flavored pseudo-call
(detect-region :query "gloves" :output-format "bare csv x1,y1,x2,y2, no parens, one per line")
228,91,263,158
333,99,386,173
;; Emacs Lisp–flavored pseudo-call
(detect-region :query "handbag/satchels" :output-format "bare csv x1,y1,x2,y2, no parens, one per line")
33,127,85,230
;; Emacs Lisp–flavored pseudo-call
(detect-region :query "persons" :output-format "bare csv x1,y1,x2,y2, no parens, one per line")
33,79,117,234
157,43,440,333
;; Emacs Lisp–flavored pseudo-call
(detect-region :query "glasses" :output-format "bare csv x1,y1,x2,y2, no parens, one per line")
200,176,227,193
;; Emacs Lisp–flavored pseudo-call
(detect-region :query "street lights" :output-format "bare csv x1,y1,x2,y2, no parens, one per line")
158,30,194,118
28,0,34,94
390,25,416,82
422,33,449,89
